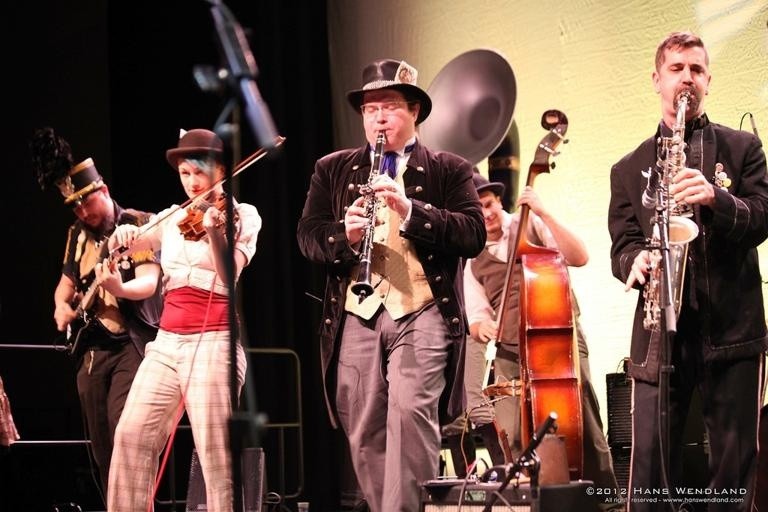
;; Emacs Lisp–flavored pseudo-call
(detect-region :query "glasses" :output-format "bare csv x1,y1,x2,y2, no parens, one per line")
359,98,411,125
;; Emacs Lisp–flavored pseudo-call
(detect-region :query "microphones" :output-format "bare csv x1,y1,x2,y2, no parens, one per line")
749,113,759,135
472,395,508,410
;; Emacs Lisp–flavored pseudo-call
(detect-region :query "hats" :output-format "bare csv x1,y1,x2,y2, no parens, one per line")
29,127,106,207
344,59,434,126
165,128,225,172
471,170,507,196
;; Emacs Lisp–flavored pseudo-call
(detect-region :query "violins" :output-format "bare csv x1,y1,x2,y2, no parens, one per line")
178,191,239,237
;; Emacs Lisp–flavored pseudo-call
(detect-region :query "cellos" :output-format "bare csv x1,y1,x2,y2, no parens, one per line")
482,109,584,481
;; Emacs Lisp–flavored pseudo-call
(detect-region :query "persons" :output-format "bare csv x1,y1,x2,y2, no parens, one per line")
608,31,765,512
53,158,163,512
106,129,261,512
462,176,623,510
298,57,485,512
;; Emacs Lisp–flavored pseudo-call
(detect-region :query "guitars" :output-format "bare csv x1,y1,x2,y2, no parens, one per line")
64,248,121,357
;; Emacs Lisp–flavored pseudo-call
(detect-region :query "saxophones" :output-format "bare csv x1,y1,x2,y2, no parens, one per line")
642,90,701,331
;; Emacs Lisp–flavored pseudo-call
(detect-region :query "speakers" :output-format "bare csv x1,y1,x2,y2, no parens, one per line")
185,446,269,512
605,371,715,512
